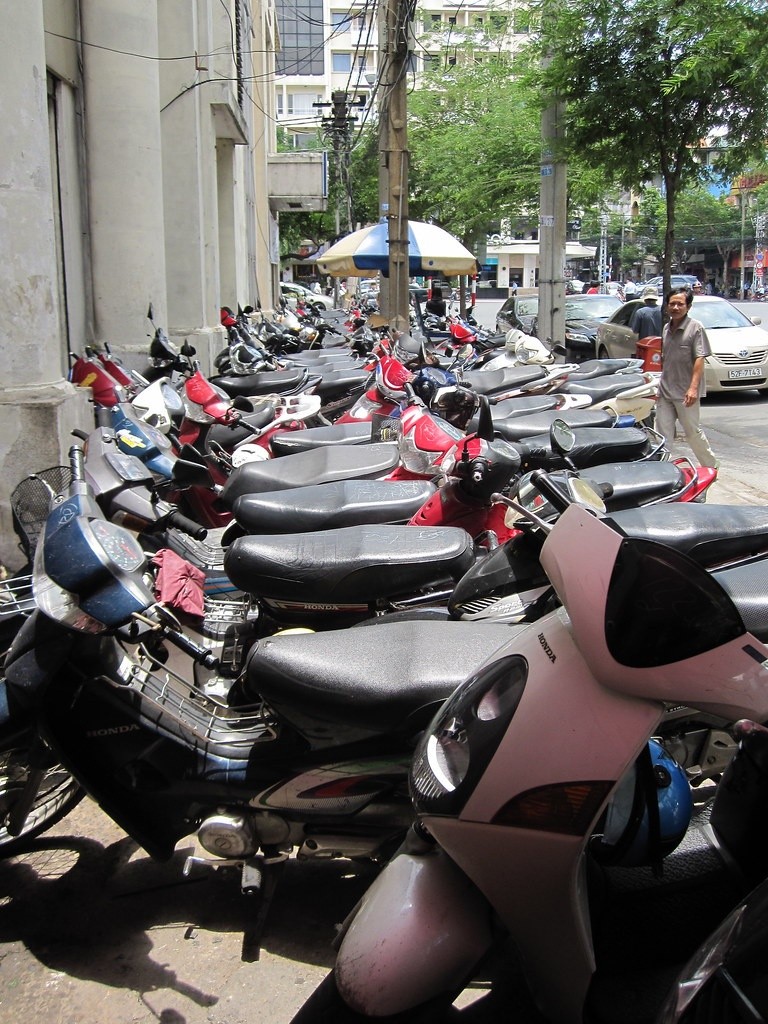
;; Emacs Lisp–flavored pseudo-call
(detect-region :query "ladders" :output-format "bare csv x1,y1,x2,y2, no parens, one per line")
598,226,607,295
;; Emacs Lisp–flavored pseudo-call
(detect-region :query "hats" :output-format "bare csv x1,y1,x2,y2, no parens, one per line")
692,282,702,287
640,287,661,300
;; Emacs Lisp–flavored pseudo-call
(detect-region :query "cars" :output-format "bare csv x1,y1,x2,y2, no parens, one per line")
495,293,539,340
633,275,703,297
561,292,622,367
595,294,768,398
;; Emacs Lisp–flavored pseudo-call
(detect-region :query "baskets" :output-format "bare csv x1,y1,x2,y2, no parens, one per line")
11,465,71,568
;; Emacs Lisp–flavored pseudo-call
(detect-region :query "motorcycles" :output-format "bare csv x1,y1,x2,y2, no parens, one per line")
1,279,768,1024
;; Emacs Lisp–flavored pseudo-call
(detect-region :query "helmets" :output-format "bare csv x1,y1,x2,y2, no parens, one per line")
611,737,693,869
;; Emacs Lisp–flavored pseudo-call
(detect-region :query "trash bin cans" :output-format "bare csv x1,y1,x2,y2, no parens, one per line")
636,335,662,399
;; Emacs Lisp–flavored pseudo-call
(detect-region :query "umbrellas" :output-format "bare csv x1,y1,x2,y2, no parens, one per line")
316,217,477,278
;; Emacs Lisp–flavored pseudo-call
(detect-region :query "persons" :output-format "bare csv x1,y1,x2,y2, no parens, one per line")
655,288,720,481
692,283,704,295
704,281,768,301
624,277,637,301
581,281,595,293
447,285,460,315
426,287,447,330
306,280,322,295
586,281,600,294
512,281,518,296
632,287,663,339
